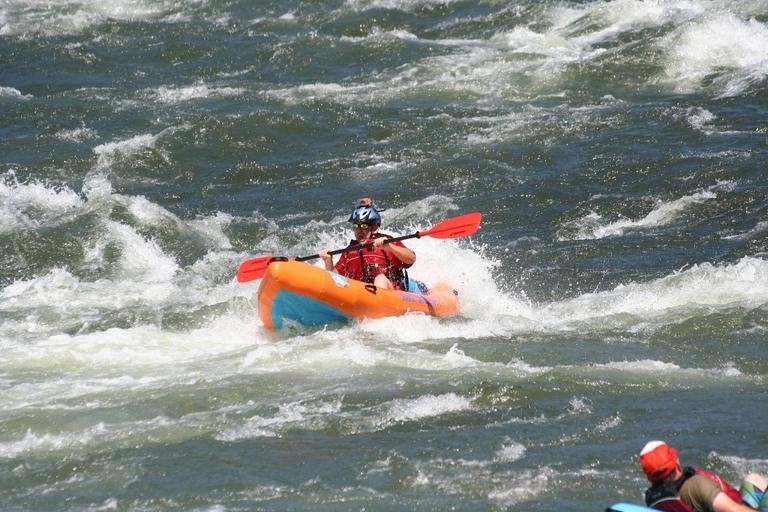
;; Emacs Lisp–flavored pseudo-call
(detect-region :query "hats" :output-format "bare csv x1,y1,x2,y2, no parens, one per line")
639,440,678,482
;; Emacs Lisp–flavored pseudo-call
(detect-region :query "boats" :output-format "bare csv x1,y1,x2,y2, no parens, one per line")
258,261,459,329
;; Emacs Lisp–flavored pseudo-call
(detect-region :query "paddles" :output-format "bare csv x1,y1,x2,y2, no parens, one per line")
237,213,482,283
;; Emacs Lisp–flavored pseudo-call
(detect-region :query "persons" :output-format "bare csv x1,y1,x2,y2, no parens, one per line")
319,197,416,290
640,440,768,512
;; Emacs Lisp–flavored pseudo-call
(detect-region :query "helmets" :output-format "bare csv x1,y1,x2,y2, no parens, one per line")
347,205,381,228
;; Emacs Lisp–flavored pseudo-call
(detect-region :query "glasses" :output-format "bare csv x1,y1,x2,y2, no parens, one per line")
350,222,372,229
